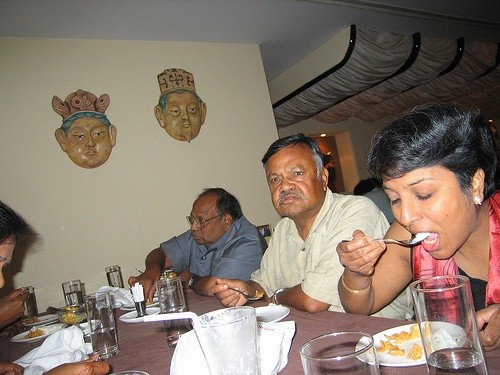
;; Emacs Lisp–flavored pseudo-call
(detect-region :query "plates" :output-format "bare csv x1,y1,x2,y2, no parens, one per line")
119,293,168,311
354,321,467,367
20,314,60,327
79,319,101,335
254,305,290,323
11,323,68,343
119,307,161,324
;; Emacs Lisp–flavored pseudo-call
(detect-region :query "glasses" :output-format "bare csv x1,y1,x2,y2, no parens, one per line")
187,210,229,228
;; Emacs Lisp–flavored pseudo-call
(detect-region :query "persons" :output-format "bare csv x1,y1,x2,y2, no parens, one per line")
353,178,394,225
154,69,207,141
130,188,267,303
52,88,117,168
0,200,27,375
210,133,415,321
336,101,500,352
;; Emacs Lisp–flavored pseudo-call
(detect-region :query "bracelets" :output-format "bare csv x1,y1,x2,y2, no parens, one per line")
340,274,372,295
273,289,285,304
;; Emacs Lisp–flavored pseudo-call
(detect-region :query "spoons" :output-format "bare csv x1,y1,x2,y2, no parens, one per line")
215,282,261,301
341,233,429,248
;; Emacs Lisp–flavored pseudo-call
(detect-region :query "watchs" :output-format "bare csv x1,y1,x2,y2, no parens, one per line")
188,274,196,287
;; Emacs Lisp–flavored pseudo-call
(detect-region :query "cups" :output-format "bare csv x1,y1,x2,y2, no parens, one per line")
190,305,261,375
83,290,120,360
299,331,380,375
58,304,88,326
134,299,149,318
155,276,188,347
408,274,489,375
61,279,83,307
20,286,38,319
104,265,124,288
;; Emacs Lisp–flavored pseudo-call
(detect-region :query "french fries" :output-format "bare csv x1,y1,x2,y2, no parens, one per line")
373,322,434,359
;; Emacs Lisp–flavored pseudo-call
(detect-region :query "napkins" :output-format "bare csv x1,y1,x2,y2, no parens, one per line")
95,285,134,307
169,320,295,375
12,325,93,375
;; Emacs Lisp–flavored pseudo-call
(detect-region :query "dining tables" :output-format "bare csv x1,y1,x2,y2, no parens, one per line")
0,282,500,375
